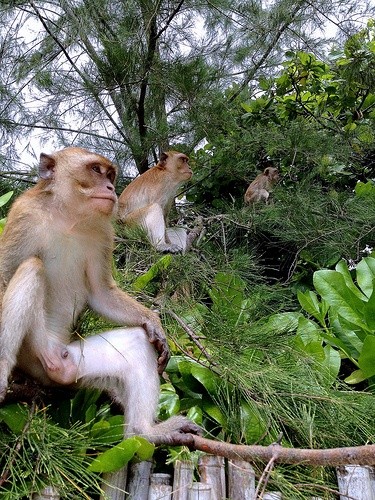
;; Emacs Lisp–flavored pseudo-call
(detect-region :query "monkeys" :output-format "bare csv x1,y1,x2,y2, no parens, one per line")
243,167,280,206
0,145,199,447
114,151,193,253
